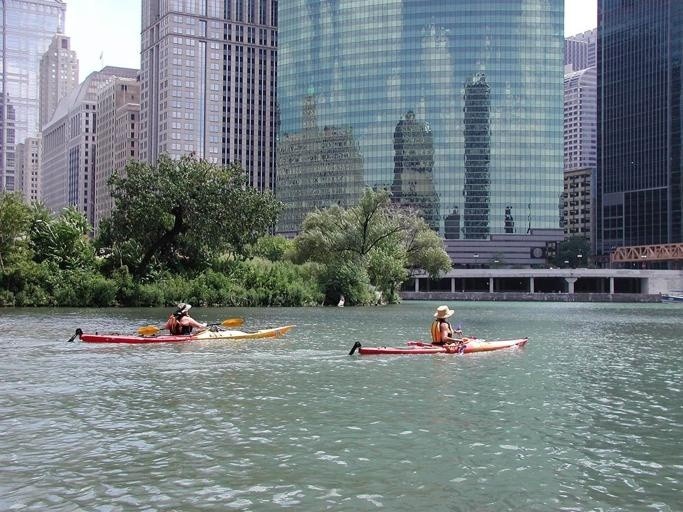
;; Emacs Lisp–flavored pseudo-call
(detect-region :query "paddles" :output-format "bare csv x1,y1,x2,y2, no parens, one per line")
456,322,466,354
138,318,244,335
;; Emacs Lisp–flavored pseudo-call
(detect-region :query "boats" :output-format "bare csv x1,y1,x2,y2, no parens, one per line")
348,335,528,359
660,290,683,304
67,325,298,345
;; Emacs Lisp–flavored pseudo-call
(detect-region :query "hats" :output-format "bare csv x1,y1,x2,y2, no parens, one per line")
176,302,192,315
433,305,455,319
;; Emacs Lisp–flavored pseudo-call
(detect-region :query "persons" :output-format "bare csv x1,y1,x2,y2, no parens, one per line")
430,304,466,344
168,303,207,335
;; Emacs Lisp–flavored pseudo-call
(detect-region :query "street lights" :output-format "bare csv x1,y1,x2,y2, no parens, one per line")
471,253,649,270
